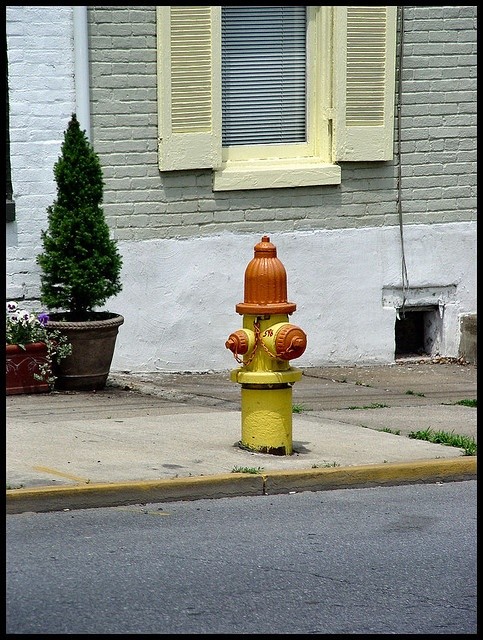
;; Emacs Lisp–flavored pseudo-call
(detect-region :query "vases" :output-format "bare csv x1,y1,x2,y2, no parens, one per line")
3,342,55,395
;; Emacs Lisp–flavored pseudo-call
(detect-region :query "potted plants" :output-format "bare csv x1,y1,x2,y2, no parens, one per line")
36,113,124,391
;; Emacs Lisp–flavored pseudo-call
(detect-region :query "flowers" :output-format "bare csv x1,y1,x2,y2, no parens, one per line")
4,301,73,388
